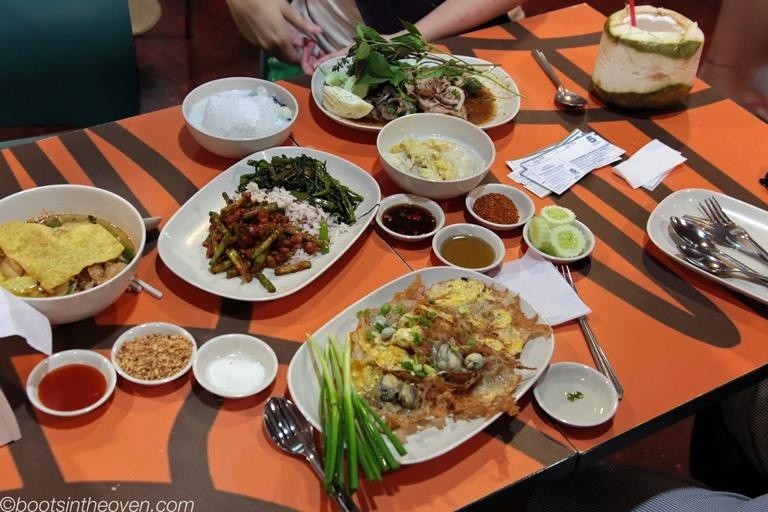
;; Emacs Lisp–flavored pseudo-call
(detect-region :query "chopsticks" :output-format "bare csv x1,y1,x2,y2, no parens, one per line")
129,276,163,299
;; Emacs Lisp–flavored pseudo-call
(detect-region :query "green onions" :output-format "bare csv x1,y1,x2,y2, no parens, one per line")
303,330,408,500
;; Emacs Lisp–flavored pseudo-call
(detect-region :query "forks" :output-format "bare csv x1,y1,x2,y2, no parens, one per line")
682,215,767,263
697,193,768,263
555,265,624,402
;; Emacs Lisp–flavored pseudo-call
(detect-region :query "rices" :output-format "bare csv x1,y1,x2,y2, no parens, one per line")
188,84,293,139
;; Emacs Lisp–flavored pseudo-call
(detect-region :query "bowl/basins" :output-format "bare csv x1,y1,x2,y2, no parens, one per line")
0,184,147,324
181,77,299,159
376,113,496,200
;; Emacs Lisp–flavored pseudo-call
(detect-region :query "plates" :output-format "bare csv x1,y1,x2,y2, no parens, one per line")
374,192,446,242
431,222,506,273
311,54,521,132
527,217,596,265
465,183,535,231
156,146,382,302
109,321,197,386
193,332,278,399
25,350,117,417
287,266,555,466
533,361,619,429
646,188,767,305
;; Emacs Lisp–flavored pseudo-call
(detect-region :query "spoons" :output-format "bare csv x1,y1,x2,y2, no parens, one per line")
678,244,767,280
531,48,587,107
670,216,767,287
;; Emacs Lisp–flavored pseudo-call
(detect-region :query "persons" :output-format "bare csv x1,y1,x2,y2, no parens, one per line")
0,0,322,142
300,0,531,78
457,459,767,511
